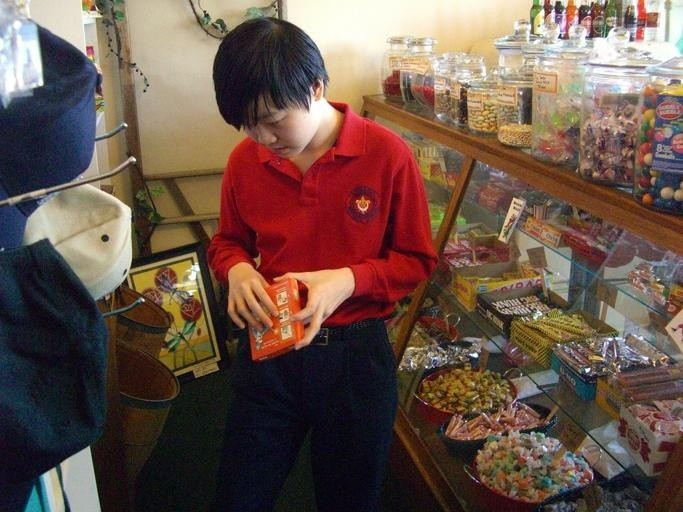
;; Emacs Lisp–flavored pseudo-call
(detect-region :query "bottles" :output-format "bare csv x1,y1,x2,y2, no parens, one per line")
631,57,682,217
578,58,661,190
432,52,468,124
411,38,440,110
554,27,592,52
530,49,596,167
530,0,660,42
496,42,554,147
466,65,506,138
448,55,487,129
383,37,406,105
494,21,542,46
593,26,656,59
399,38,423,108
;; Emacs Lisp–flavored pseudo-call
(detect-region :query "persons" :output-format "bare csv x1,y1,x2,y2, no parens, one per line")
202,18,437,510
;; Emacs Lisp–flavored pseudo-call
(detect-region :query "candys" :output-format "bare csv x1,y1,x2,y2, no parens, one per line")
379,68,682,512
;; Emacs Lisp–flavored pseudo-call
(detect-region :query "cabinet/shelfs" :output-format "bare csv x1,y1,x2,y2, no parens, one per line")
27,0,115,197
359,95,683,512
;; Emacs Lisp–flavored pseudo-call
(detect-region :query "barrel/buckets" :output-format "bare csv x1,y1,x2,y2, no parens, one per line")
118,336,181,487
115,284,171,358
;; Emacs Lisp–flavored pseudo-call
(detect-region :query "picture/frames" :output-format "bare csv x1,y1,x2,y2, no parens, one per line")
119,241,231,389
